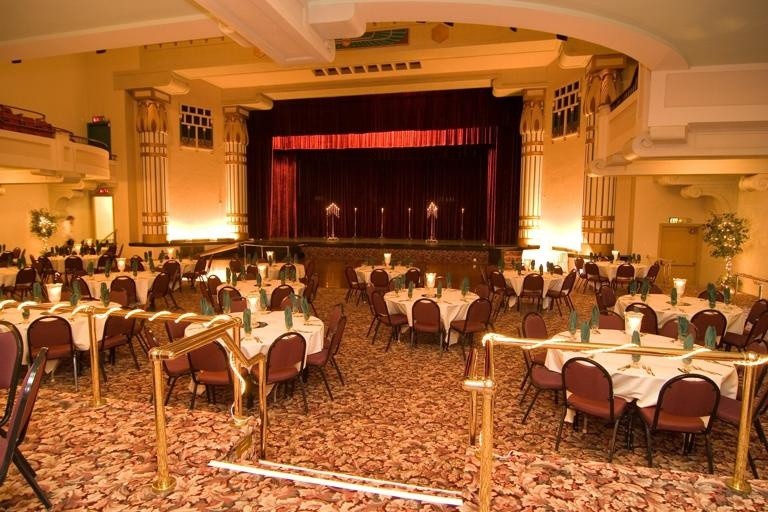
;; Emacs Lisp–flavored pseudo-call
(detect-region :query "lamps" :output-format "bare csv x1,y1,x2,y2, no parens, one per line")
247,295,258,326
426,272,436,287
383,253,391,263
672,277,686,296
611,250,619,259
625,312,643,337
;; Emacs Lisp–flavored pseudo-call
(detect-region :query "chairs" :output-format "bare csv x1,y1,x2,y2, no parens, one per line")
97,255,112,272
725,309,768,350
300,261,315,282
714,386,768,480
586,261,609,293
271,285,294,311
406,269,420,286
158,260,180,309
1,347,52,512
30,255,35,269
543,269,576,316
345,266,369,306
281,264,296,280
130,254,143,272
630,374,720,474
247,265,257,280
226,297,247,313
64,257,82,274
280,294,307,312
475,284,490,299
38,256,58,283
314,303,344,367
18,249,25,269
13,247,21,258
597,310,625,327
371,290,408,353
218,288,242,313
574,257,588,291
518,273,544,312
445,298,492,363
555,357,634,464
743,299,767,326
147,272,170,308
488,288,506,327
97,309,140,383
3,266,37,300
553,266,561,274
516,328,563,424
164,306,187,342
520,311,549,392
206,286,218,313
410,298,445,359
1,320,37,479
371,270,389,290
691,309,725,350
250,332,309,412
143,326,191,407
2,252,12,266
70,276,93,301
207,273,221,293
188,341,250,410
126,292,153,359
28,314,78,395
365,282,396,340
659,319,698,339
230,258,247,280
182,254,214,290
300,272,319,317
290,315,347,401
490,270,519,313
113,242,124,267
110,277,136,309
612,264,635,291
625,302,657,333
479,263,491,284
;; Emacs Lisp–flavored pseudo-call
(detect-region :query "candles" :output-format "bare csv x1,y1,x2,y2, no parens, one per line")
266,251,273,261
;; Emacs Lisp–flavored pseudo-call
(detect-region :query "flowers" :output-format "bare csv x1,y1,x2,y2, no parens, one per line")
689,207,750,297
24,208,59,251
